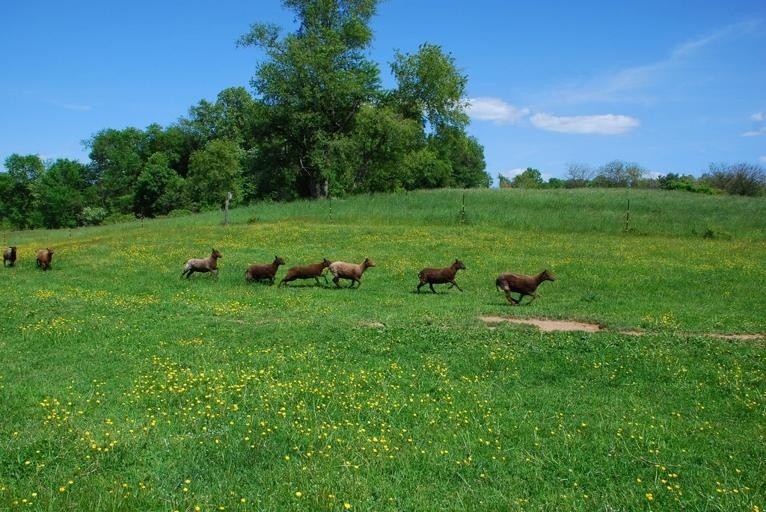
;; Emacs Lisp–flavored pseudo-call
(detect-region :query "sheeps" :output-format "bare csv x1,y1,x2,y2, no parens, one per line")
180,248,223,280
416,258,467,294
325,256,376,289
244,256,285,286
278,258,332,287
495,268,554,305
3,246,17,267
36,247,54,271
